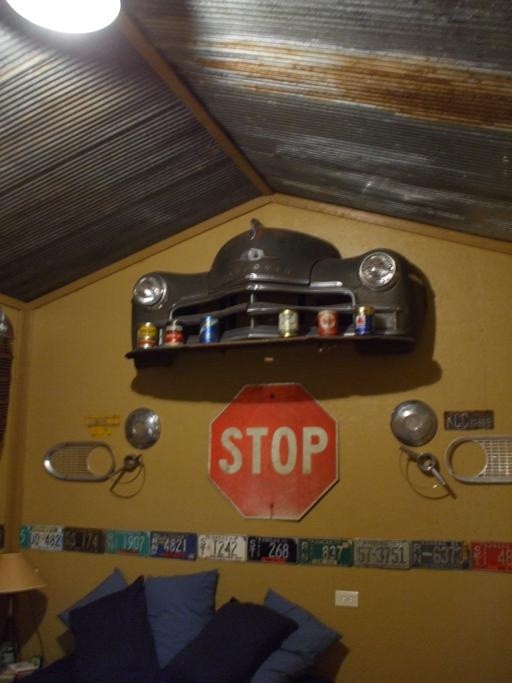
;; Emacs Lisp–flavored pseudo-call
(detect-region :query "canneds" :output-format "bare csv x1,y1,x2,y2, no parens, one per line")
199,316,220,343
137,322,158,349
317,309,339,336
279,309,299,337
163,319,183,345
354,306,375,335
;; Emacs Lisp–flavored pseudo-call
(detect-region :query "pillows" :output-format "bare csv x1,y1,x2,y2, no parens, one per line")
56,567,131,628
252,587,342,681
72,575,158,683
163,601,299,682
144,569,219,667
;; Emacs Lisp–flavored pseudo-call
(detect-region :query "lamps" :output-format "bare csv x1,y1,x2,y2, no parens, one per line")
1,552,49,675
